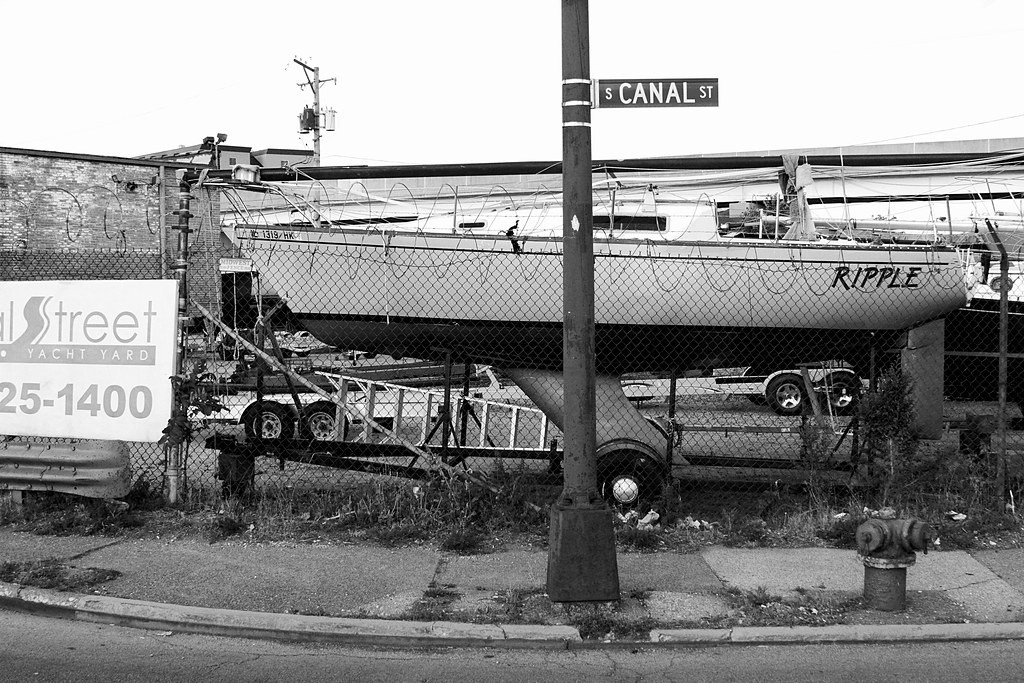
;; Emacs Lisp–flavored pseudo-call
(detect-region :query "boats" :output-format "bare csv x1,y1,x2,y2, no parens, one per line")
218,198,969,374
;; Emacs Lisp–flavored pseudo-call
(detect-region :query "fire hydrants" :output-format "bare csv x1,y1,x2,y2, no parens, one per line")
855,509,935,612
